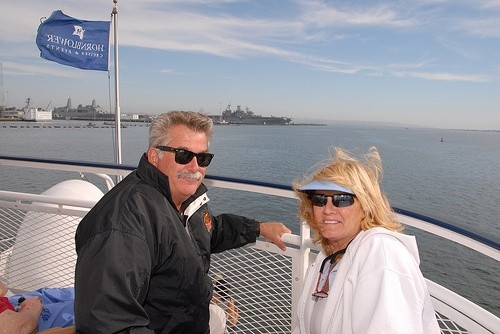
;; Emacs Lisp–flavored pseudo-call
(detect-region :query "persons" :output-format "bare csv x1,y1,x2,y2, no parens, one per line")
74,111,292,334
208,271,238,334
292,146,441,334
0,281,75,334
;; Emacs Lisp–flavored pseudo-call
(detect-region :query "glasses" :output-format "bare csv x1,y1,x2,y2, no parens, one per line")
312,249,346,297
310,194,356,208
156,146,214,167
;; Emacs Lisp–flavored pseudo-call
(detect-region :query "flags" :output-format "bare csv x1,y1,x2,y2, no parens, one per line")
36,10,113,71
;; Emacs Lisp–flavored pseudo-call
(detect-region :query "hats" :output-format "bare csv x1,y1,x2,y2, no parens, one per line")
296,180,355,194
